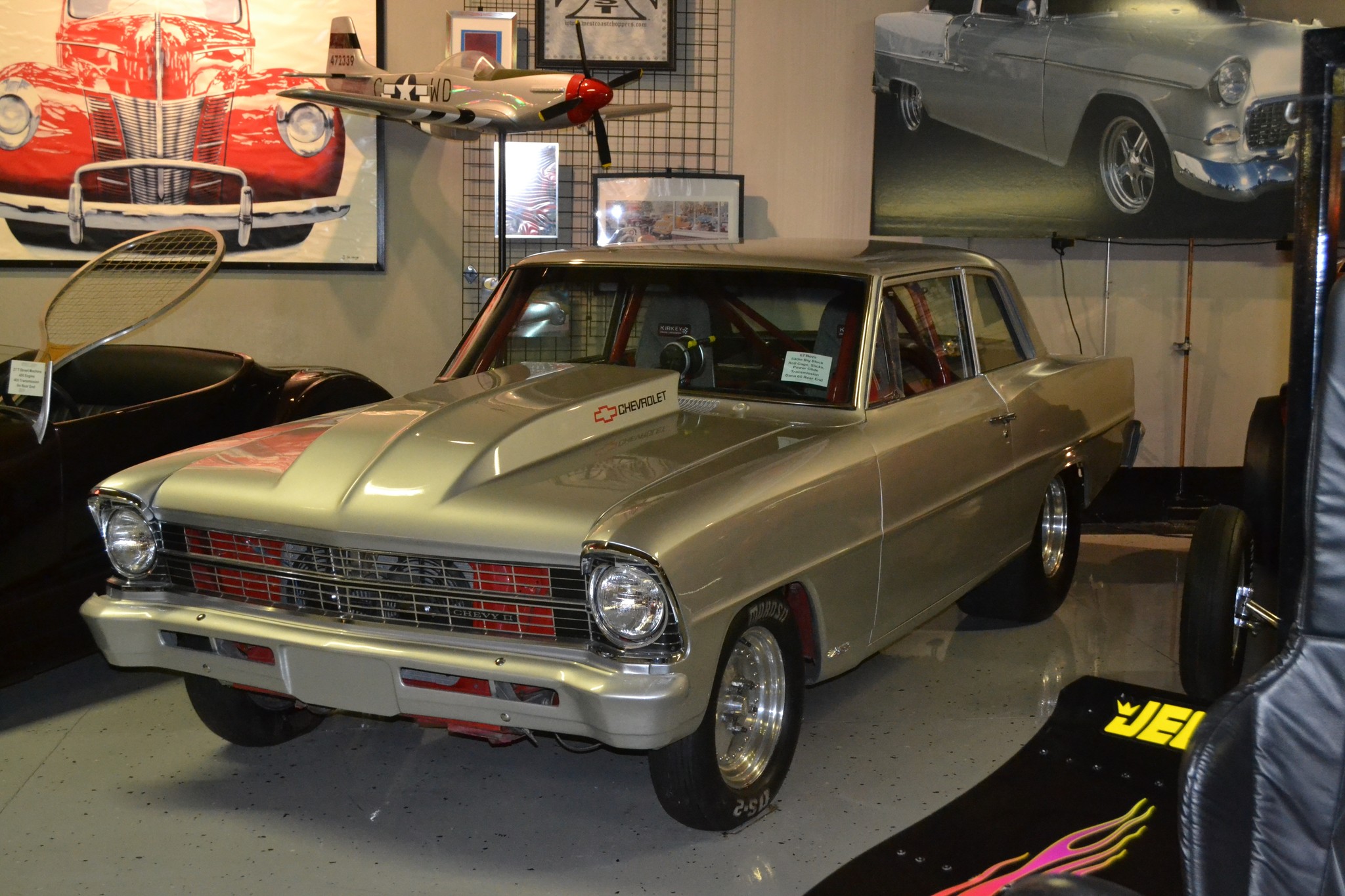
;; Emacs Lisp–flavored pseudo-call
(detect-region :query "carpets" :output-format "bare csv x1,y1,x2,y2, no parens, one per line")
803,680,1207,896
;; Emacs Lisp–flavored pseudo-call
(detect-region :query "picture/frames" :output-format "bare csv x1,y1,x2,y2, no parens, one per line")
590,171,746,246
491,140,559,239
534,1,678,75
441,9,518,69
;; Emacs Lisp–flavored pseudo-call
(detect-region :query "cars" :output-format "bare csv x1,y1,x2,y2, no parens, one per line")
872,0,1331,223
0,344,393,691
79,244,1147,833
0,0,351,255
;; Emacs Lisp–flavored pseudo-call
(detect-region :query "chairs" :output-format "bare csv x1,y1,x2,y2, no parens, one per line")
634,291,721,391
802,293,905,406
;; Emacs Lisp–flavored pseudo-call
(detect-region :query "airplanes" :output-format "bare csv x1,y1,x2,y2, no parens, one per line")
275,15,673,169
